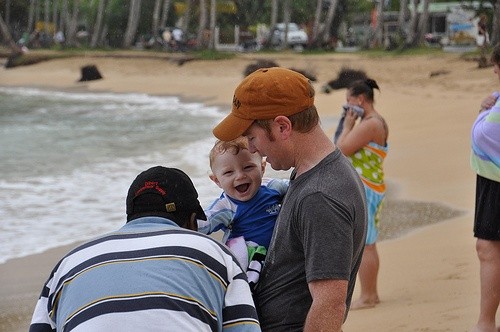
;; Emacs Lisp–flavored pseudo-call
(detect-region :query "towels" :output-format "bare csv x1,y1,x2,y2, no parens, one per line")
334,104,364,144
469,90,500,182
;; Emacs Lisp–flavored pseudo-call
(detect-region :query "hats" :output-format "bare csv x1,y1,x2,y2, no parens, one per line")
212,67,314,141
126,166,208,222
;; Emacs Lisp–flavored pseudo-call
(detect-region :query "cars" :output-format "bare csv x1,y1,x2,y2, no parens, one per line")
272,22,309,48
